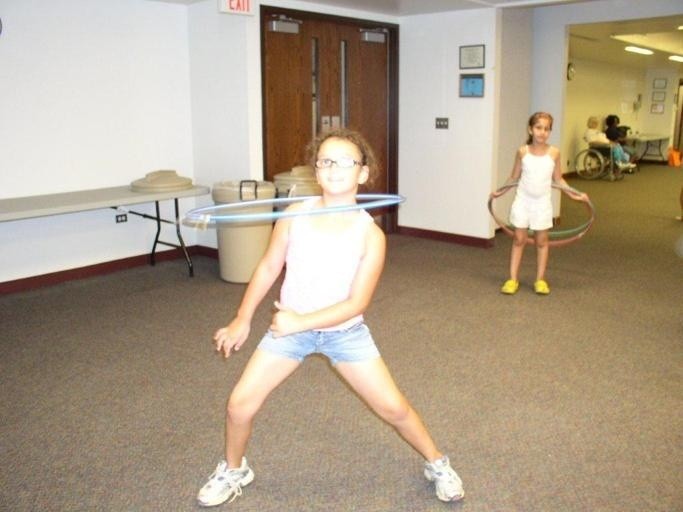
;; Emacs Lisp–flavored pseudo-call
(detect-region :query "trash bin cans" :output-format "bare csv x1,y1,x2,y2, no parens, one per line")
212,166,323,285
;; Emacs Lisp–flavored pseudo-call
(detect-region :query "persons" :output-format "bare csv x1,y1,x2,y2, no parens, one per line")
491,111,589,294
195,133,465,507
583,116,629,167
605,114,638,163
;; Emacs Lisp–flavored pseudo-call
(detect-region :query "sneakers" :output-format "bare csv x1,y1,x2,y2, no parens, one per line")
196,456,256,508
500,280,521,296
423,453,467,504
533,279,551,297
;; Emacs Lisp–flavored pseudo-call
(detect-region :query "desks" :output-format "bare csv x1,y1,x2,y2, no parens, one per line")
1,186,210,277
616,135,669,166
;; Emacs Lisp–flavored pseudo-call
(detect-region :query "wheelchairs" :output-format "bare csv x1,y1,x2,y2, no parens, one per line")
573,142,625,183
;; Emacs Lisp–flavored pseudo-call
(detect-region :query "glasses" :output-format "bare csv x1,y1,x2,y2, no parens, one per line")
312,157,362,171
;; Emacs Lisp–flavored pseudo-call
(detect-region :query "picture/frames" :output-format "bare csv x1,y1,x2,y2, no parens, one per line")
650,103,665,114
458,44,485,70
651,91,665,102
652,78,667,89
